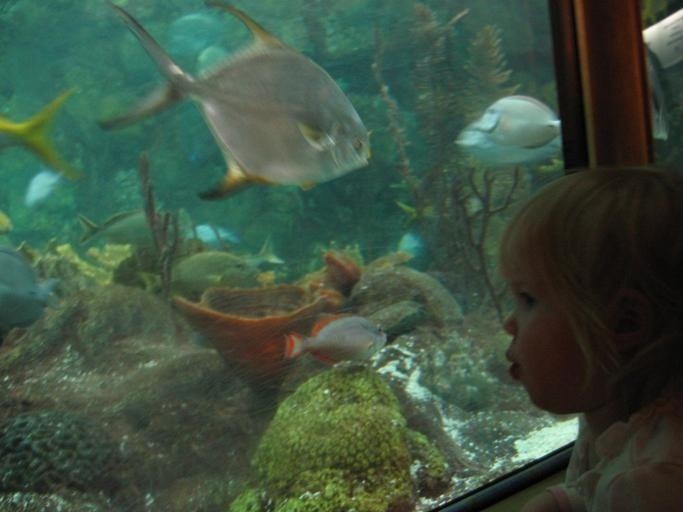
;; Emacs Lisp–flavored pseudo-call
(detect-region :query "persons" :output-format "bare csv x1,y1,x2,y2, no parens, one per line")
499,162,682,512
